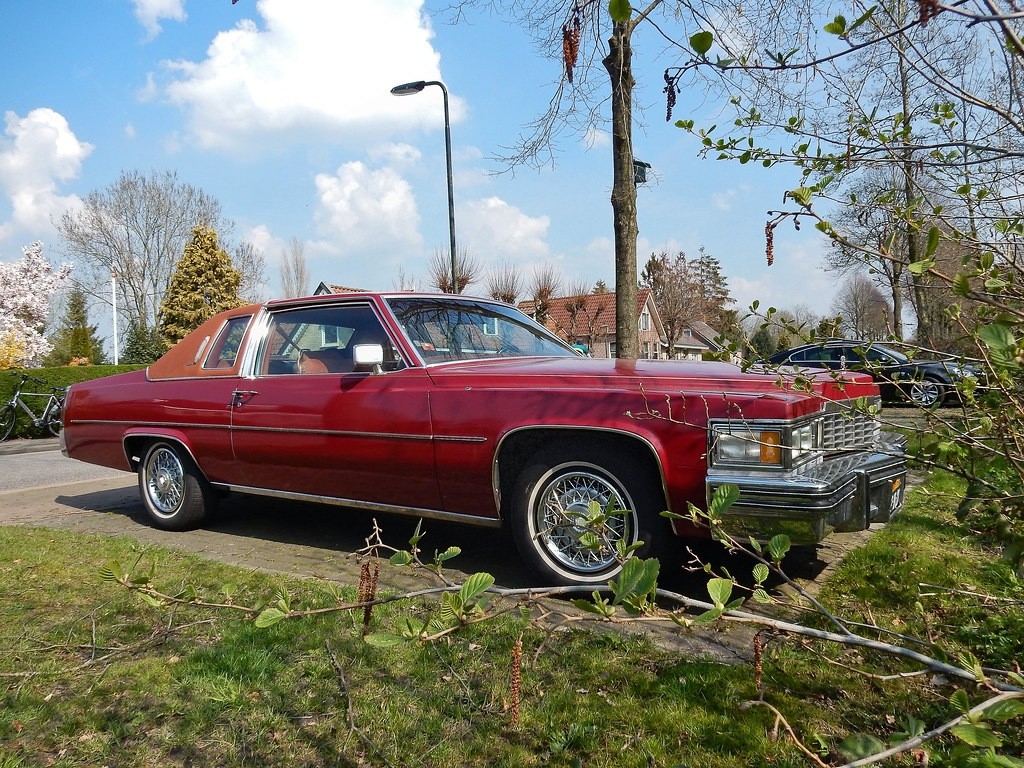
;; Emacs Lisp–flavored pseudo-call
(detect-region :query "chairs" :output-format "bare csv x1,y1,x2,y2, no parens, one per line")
216,328,395,374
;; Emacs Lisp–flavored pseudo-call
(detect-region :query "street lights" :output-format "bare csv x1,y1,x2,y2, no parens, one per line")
390,79,465,362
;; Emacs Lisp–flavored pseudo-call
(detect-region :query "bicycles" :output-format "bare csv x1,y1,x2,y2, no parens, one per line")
1,371,67,445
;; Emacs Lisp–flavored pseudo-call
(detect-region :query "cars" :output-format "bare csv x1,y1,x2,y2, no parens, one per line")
58,289,911,601
748,338,988,409
